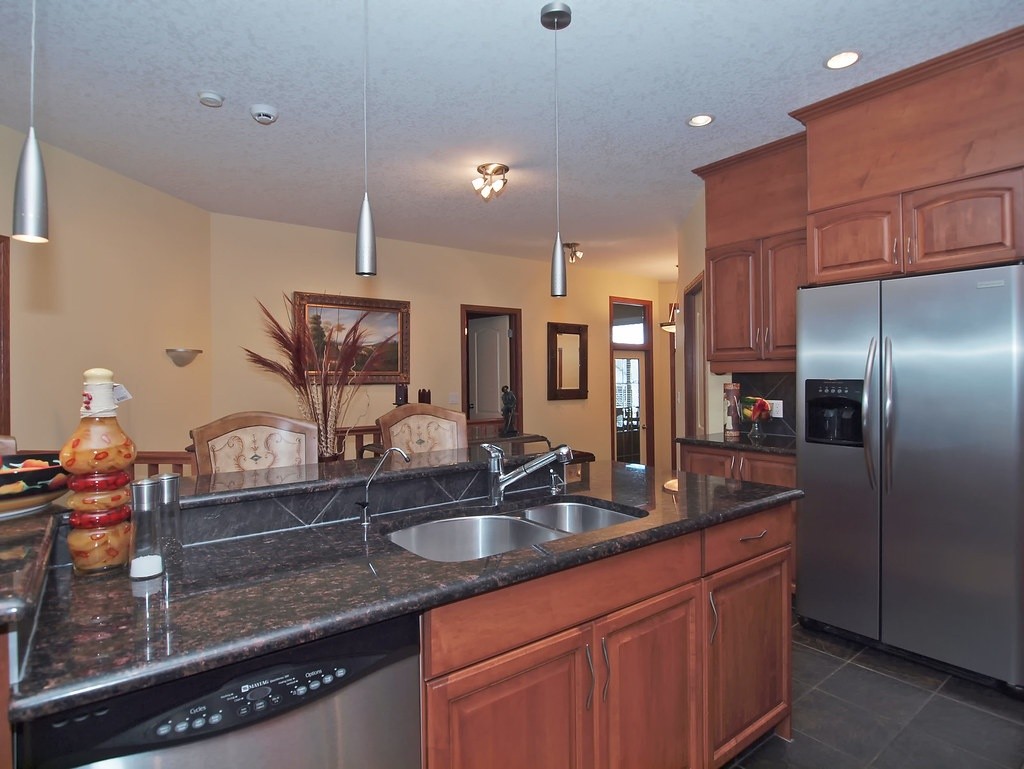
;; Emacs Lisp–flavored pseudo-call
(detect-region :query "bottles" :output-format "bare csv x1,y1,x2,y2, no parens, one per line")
58,367,136,572
128,479,165,582
150,472,183,566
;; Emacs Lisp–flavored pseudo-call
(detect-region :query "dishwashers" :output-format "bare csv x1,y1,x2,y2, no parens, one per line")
14,614,420,769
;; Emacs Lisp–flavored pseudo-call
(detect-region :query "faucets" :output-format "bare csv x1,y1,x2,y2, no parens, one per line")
479,443,573,501
354,447,411,525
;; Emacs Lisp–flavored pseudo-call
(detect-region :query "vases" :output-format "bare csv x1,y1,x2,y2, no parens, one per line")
748,420,767,443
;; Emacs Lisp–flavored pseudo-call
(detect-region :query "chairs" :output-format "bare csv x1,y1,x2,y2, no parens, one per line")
357,402,470,459
189,411,318,471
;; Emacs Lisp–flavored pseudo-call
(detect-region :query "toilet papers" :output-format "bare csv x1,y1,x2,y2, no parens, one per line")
723,382,741,437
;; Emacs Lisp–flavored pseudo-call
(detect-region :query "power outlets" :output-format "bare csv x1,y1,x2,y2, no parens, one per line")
765,400,783,417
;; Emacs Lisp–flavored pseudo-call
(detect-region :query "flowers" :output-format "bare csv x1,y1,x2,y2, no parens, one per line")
746,399,770,422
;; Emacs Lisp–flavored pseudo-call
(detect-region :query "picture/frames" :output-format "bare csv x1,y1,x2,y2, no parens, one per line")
290,288,411,387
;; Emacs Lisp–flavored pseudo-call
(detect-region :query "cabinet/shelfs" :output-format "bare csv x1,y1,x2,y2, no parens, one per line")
803,165,1024,288
702,227,807,366
701,504,801,769
420,532,703,769
677,444,796,488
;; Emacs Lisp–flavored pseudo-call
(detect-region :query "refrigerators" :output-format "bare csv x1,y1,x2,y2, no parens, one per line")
795,260,1024,699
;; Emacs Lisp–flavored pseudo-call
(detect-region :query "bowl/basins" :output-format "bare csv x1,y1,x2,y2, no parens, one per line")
0,452,71,521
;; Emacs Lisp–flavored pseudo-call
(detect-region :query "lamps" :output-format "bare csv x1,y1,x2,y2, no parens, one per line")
660,263,678,332
540,2,572,298
471,162,510,200
562,241,585,264
165,347,203,367
355,0,378,277
13,0,49,244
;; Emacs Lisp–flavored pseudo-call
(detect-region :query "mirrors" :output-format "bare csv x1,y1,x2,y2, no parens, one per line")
546,321,589,402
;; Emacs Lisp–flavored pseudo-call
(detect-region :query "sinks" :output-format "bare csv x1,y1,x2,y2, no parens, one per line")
386,517,571,562
523,503,641,534
